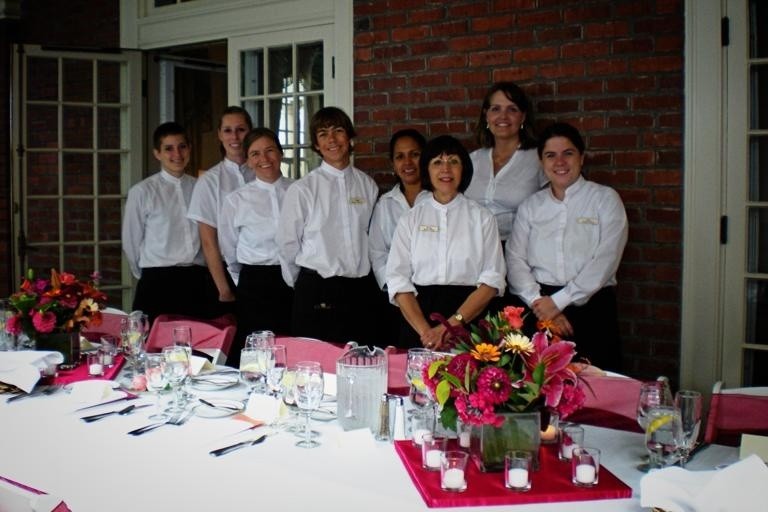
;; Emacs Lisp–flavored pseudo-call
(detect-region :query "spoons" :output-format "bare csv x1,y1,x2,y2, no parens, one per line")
209,432,270,457
79,404,136,422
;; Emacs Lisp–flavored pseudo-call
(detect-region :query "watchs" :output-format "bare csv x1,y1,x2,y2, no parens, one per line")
451,310,468,331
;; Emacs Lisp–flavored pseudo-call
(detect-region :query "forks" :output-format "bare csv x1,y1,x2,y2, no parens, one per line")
126,411,182,434
133,410,195,437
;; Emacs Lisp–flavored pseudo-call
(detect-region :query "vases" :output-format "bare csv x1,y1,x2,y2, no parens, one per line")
475,409,547,473
33,327,81,371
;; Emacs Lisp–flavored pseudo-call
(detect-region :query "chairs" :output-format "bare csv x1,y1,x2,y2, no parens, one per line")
80,307,142,352
379,345,416,399
567,370,665,434
704,381,767,449
260,330,359,375
143,315,236,367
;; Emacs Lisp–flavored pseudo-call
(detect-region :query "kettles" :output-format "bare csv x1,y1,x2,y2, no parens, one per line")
335,345,389,438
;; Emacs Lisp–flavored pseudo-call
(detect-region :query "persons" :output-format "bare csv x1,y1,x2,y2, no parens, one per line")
187,107,251,322
369,130,423,347
387,136,506,353
121,122,221,324
464,82,547,246
217,128,294,335
509,123,627,372
279,107,378,348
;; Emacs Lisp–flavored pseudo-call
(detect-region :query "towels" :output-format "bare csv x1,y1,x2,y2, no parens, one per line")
0,348,64,395
640,450,768,512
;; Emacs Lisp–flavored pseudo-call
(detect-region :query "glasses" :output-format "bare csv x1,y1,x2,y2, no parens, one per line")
427,157,462,169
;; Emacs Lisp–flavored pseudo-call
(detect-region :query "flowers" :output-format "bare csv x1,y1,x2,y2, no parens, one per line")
6,267,108,350
422,305,600,431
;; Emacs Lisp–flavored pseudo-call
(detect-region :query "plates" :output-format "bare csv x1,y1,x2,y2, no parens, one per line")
192,399,245,420
191,374,240,391
62,379,120,390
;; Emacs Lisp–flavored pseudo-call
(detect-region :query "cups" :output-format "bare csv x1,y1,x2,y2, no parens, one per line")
100,336,115,357
672,390,704,470
644,407,684,469
144,352,173,423
86,352,105,377
172,327,192,343
440,450,470,492
99,345,115,370
120,311,149,378
411,413,436,448
159,345,198,415
635,382,670,474
244,329,275,349
120,322,147,392
456,416,471,448
238,347,267,394
421,432,447,472
504,450,533,493
293,360,325,449
559,424,584,462
540,412,561,443
571,445,601,489
261,346,289,401
405,347,433,387
